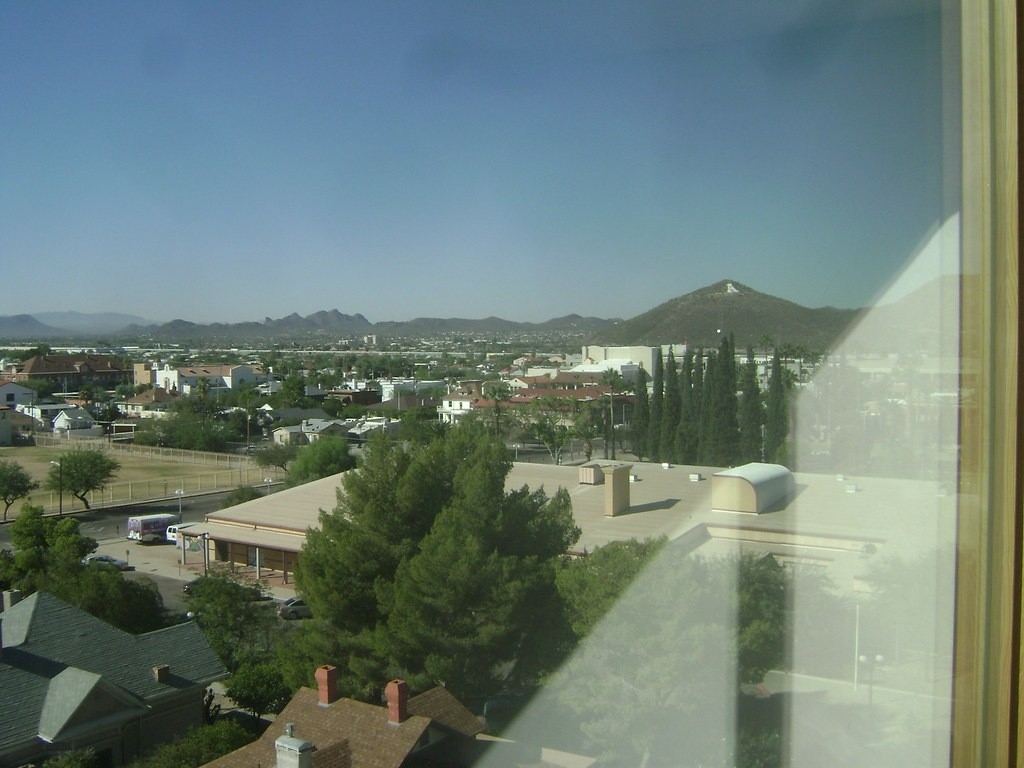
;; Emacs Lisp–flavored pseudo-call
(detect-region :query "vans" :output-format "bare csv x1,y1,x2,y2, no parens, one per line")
167,521,202,544
126,514,178,545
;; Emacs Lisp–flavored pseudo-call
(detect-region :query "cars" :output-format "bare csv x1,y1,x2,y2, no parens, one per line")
277,595,314,620
